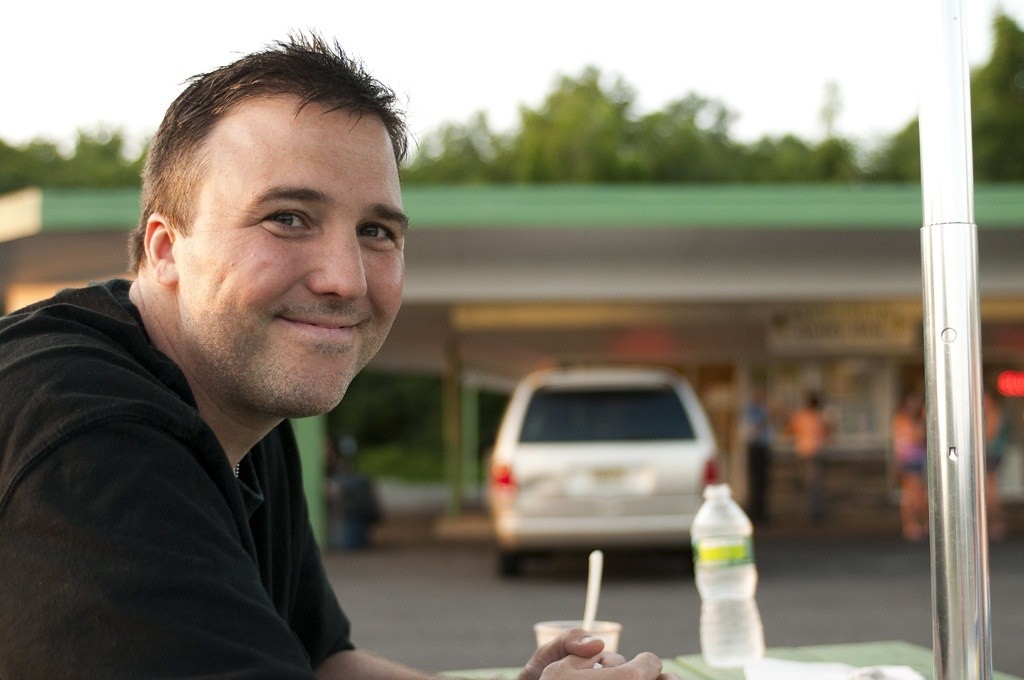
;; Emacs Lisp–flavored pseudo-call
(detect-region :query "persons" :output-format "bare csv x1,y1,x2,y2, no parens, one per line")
779,388,839,516
738,368,771,520
889,389,931,540
0,30,673,680
983,388,1005,533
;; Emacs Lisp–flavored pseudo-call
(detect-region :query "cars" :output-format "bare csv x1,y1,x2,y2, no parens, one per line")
488,362,723,578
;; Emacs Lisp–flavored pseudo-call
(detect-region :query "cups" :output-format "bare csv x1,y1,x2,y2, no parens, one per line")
534,620,622,669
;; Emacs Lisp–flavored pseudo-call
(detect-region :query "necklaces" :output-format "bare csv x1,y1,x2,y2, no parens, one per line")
235,464,239,478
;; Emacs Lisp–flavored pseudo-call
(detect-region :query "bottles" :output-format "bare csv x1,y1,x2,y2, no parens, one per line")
690,483,765,668
